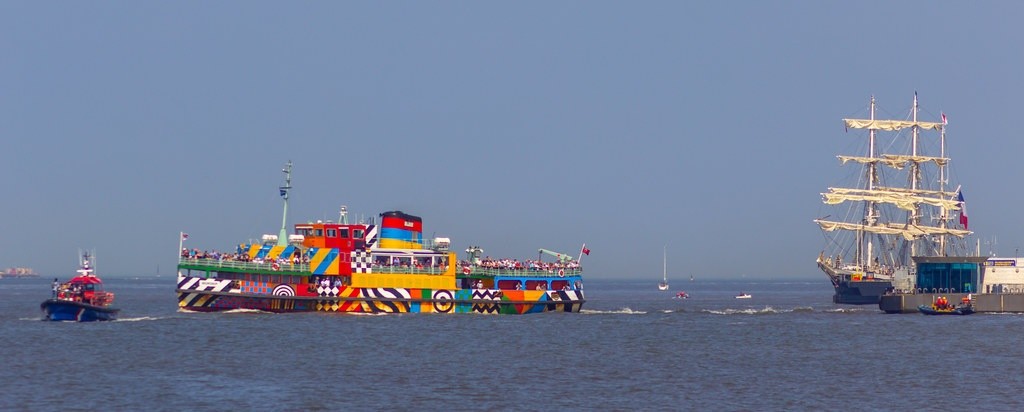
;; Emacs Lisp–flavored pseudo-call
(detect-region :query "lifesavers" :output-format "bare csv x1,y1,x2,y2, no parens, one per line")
243,253,250,259
464,268,470,275
559,270,564,277
272,263,279,271
105,293,113,302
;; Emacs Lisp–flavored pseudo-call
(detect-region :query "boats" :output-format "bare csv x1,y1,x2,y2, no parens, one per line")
38,248,121,323
734,293,751,299
917,294,977,316
174,158,590,314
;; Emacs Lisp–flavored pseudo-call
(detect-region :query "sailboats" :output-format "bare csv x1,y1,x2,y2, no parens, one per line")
812,89,982,305
657,246,670,291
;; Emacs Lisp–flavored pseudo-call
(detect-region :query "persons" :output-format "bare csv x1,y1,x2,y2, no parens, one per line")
931,295,963,311
883,285,916,295
181,248,311,271
376,255,579,276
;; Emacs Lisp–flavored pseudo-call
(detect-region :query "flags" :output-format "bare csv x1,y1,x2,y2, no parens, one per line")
955,191,968,231
582,248,590,256
280,189,286,196
